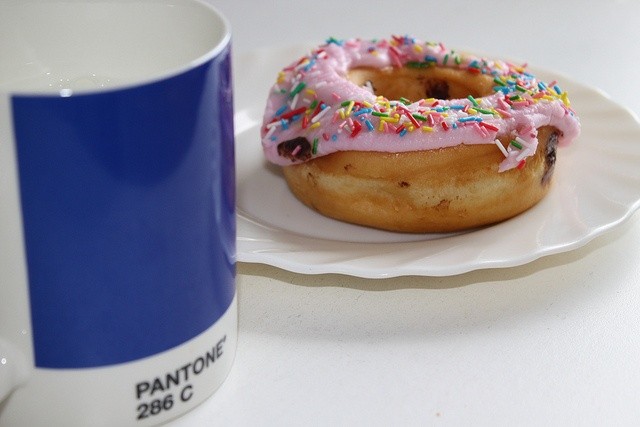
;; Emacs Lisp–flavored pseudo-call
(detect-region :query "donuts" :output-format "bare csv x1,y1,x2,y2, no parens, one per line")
261,29,581,236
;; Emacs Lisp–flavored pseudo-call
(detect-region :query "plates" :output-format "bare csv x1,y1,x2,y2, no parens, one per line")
233,69,637,278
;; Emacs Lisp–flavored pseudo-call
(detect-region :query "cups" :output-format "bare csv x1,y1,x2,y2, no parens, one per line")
1,1,237,426
